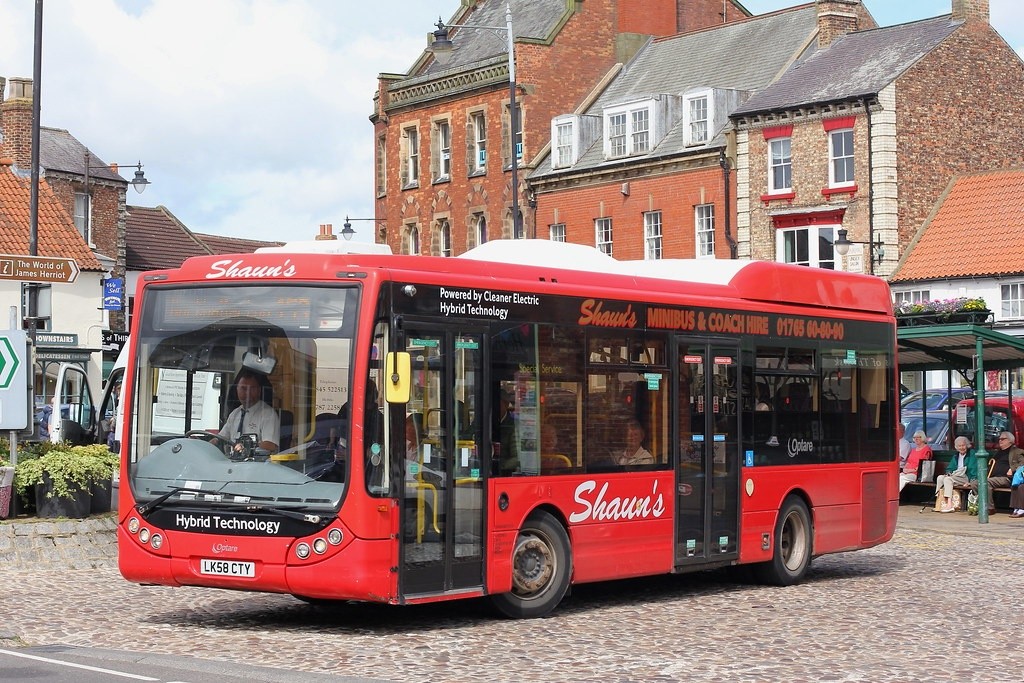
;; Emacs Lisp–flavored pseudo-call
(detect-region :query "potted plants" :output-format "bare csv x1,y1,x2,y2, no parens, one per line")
0,439,121,518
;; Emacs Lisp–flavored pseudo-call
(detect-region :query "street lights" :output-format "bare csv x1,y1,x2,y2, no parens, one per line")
424,17,519,239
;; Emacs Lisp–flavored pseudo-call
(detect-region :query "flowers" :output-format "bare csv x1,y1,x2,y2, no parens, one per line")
893,295,988,320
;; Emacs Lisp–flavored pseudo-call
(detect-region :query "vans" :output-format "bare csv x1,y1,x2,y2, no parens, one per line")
47,319,350,448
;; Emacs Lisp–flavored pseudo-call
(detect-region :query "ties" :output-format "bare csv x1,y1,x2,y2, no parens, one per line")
237,409,249,437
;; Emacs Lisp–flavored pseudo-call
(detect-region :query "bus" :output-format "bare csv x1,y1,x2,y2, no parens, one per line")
117,238,899,620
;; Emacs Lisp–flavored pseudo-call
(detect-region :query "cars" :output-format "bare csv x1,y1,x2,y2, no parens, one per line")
545,388,577,402
950,396,1024,450
901,388,973,410
900,410,950,450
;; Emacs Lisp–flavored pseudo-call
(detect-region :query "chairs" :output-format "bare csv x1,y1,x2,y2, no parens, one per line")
932,395,942,399
275,382,869,485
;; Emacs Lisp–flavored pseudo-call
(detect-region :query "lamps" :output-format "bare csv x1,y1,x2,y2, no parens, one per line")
339,215,387,240
830,229,884,264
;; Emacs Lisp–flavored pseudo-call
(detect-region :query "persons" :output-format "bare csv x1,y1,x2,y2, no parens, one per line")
755,381,770,415
1008,484,1024,518
106,384,121,446
408,412,437,463
208,373,280,461
973,432,1024,515
39,397,53,441
612,418,655,467
935,436,977,515
328,379,378,452
470,388,565,470
900,424,933,494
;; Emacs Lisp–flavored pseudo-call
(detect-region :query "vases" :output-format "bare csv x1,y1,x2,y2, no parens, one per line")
897,309,991,327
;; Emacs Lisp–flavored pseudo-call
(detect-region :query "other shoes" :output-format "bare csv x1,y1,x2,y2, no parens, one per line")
1009,512,1024,518
989,509,996,515
941,508,955,513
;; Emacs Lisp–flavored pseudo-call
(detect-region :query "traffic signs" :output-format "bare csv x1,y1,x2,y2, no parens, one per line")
0,254,80,285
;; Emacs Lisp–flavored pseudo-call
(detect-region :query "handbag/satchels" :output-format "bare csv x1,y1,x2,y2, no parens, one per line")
935,489,962,512
967,490,979,516
915,451,937,483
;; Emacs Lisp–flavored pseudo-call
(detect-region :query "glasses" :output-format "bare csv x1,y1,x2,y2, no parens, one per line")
998,438,1007,441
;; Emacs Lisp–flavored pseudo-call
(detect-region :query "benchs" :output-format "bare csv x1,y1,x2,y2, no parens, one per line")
909,450,1011,512
